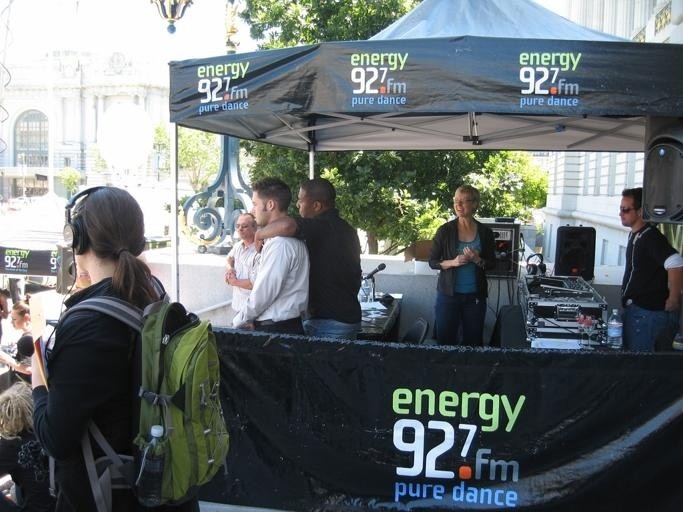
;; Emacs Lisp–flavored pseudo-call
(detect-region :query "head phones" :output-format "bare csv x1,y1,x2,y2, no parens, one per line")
63,186,146,257
526,253,546,275
374,295,394,304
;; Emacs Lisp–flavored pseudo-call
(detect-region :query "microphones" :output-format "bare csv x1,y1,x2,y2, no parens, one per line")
500,252,519,267
367,263,386,280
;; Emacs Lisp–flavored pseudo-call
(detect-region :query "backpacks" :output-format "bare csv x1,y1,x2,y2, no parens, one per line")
45,274,230,509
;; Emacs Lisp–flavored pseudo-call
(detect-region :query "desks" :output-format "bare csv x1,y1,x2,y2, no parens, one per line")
352,295,401,341
497,304,609,353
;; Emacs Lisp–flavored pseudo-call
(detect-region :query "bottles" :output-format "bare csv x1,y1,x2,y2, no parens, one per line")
135,424,165,508
605,308,623,350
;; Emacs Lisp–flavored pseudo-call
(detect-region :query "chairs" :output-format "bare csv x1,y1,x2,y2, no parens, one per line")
398,316,428,344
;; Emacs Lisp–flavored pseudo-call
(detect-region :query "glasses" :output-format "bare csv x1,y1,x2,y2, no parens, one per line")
235,224,254,229
452,197,474,204
620,206,639,214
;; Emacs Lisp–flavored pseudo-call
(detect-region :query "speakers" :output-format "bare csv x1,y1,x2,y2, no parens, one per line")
56,246,76,294
554,226,596,281
641,115,683,225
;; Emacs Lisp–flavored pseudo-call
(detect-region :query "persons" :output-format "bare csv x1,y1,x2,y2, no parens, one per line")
0,380,54,511
0,295,35,386
25,183,198,512
428,186,495,346
0,287,21,391
253,178,365,339
224,212,269,332
617,186,683,351
231,176,309,337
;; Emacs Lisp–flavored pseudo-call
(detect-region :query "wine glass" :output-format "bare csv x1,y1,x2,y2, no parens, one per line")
575,315,596,351
360,272,373,303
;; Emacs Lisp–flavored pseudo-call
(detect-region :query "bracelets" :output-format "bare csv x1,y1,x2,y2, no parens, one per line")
476,259,483,267
